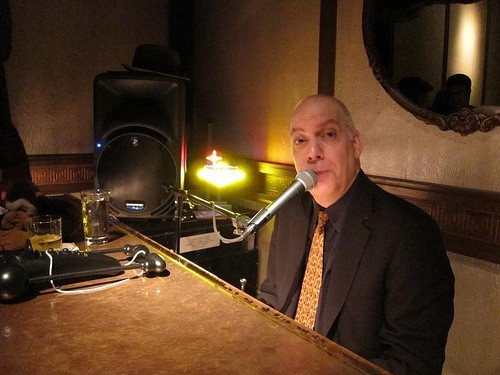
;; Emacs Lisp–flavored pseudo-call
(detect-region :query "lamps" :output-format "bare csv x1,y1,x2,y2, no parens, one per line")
196,149,248,211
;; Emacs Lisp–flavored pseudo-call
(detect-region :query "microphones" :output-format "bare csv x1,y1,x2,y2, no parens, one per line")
241,169,318,239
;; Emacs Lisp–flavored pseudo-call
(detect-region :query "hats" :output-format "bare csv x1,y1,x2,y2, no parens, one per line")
397,76,434,100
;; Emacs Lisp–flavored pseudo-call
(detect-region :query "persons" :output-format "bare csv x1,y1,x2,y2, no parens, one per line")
395,74,472,116
257,95,455,375
0,176,35,251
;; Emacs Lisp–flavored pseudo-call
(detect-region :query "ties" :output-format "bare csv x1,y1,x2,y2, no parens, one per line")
294,211,329,331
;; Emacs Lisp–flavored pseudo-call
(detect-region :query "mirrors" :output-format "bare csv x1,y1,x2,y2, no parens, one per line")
362,0,500,136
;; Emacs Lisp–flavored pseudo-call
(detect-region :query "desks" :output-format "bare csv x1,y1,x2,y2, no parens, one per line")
83,190,259,280
0,194,393,375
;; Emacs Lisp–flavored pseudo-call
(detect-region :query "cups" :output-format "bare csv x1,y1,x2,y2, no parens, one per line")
80,189,110,246
24,215,63,251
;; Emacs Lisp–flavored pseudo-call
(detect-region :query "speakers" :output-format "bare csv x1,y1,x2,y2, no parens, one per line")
92,70,185,220
